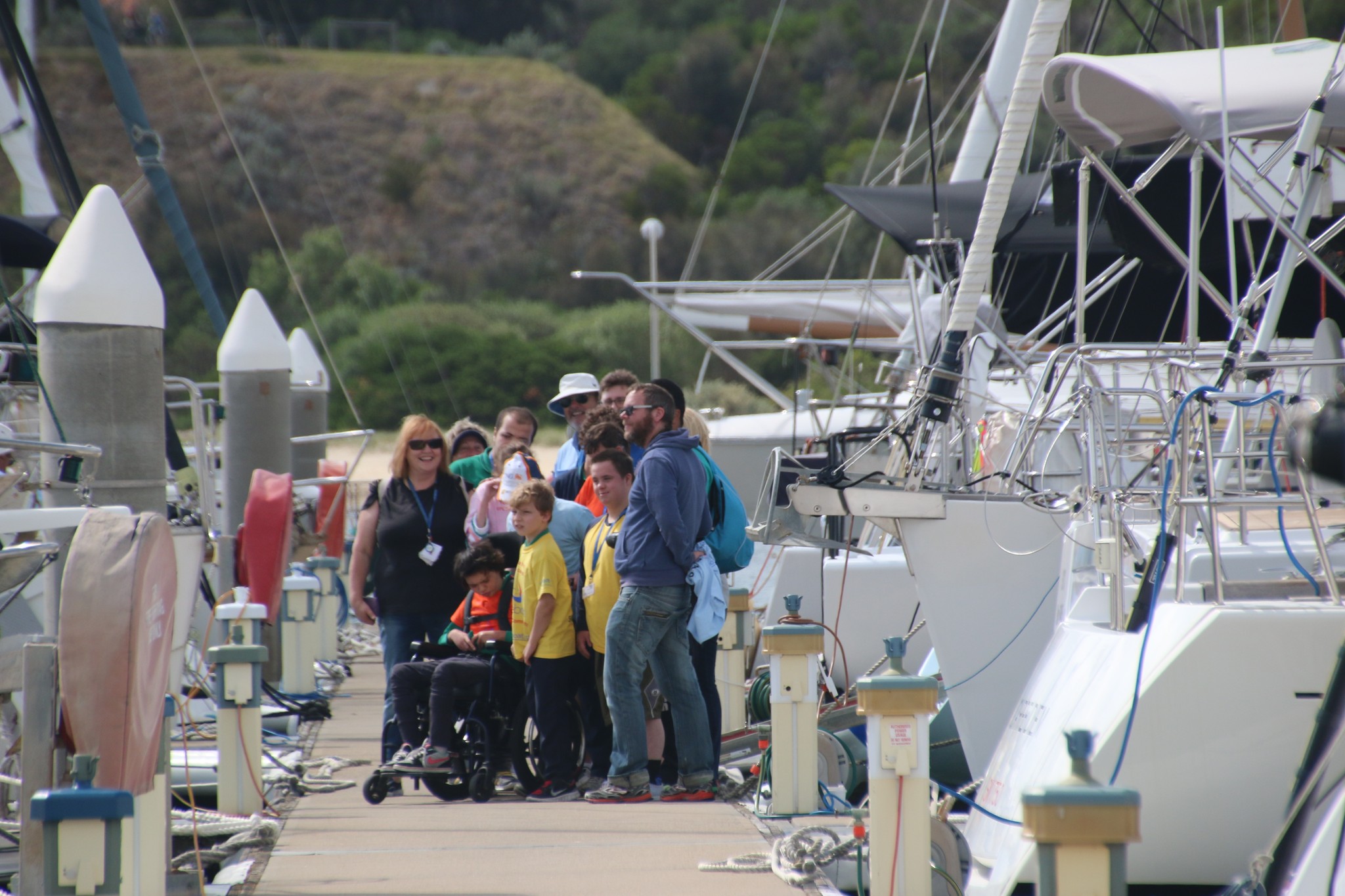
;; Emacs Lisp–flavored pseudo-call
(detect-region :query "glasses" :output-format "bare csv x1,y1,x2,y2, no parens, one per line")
559,394,592,407
408,438,442,450
621,404,652,416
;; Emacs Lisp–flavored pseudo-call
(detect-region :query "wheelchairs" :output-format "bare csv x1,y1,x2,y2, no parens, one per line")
360,637,588,806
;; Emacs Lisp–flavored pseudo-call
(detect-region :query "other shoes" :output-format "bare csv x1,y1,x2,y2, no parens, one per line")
368,778,403,796
494,771,515,791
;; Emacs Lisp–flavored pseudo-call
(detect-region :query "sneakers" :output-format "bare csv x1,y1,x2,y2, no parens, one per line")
583,783,653,802
392,738,452,773
659,780,715,802
380,743,413,773
525,781,580,801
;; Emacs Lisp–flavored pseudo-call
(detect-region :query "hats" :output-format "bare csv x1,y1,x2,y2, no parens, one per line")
547,372,600,417
452,430,487,455
497,450,543,503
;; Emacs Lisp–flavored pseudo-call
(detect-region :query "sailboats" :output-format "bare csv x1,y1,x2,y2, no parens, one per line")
0,0,378,896
568,1,1344,896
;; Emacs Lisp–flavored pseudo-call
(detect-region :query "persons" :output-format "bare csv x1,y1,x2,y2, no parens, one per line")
349,368,721,804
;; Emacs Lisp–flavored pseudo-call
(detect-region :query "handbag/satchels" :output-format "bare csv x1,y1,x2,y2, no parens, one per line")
699,448,755,574
